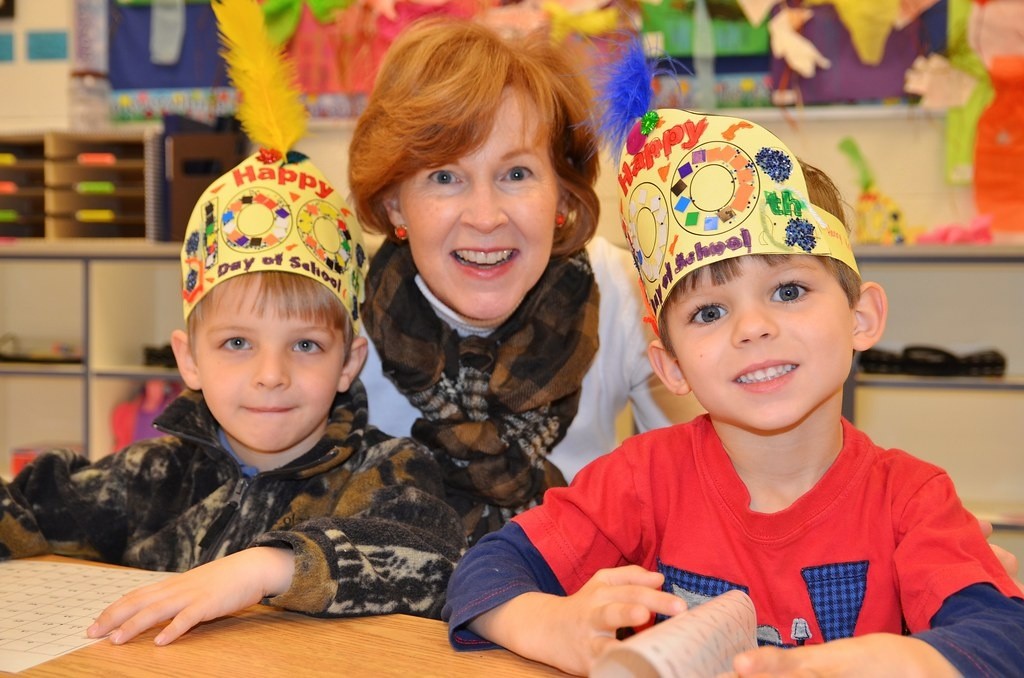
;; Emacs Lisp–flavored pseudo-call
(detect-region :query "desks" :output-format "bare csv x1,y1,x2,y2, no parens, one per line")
0,552,582,678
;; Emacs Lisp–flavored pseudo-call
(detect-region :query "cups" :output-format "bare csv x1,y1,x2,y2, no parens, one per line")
72,71,111,133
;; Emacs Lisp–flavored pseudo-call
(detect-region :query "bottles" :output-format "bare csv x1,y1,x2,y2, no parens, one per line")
855,193,883,242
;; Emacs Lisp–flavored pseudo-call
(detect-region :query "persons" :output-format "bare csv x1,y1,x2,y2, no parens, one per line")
348,15,673,547
440,108,1024,678
0,150,465,646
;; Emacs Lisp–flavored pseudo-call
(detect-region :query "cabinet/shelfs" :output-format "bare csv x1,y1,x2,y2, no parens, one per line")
0,123,165,243
840,245,1024,584
0,239,187,475
